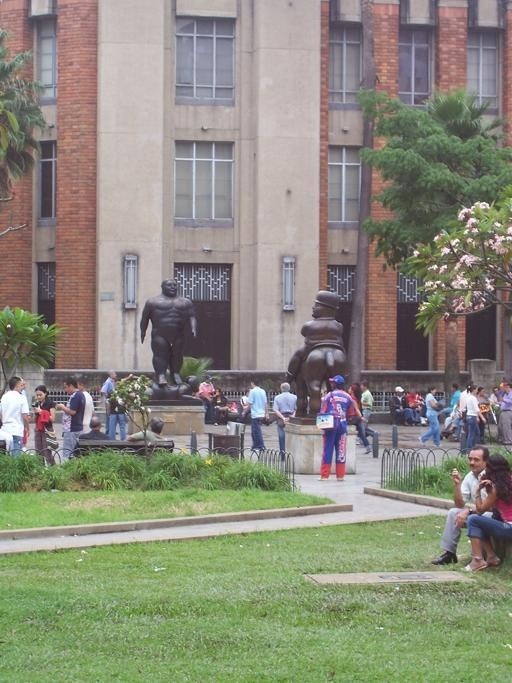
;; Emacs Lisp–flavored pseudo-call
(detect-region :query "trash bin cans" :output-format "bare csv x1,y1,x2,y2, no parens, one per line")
214,434,240,461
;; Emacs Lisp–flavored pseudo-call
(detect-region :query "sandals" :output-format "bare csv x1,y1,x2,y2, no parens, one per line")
462,554,501,574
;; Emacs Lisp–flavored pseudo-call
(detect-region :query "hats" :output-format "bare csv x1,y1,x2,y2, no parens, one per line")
396,387,404,393
330,375,344,385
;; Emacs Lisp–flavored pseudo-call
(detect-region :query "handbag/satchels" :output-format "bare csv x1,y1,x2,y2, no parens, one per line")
45,426,59,451
317,414,341,430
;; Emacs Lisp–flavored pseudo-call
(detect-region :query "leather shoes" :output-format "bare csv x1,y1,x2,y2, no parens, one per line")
432,552,457,565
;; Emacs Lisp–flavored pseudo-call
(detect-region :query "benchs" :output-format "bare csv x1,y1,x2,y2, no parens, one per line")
74,440,174,459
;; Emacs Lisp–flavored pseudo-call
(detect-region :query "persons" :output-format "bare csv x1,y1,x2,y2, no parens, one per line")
141,277,197,384
273,382,298,460
390,386,428,425
347,380,380,454
199,374,237,426
320,375,362,480
468,457,512,572
240,377,269,451
433,447,494,563
284,290,347,384
0,370,166,467
418,381,510,449
